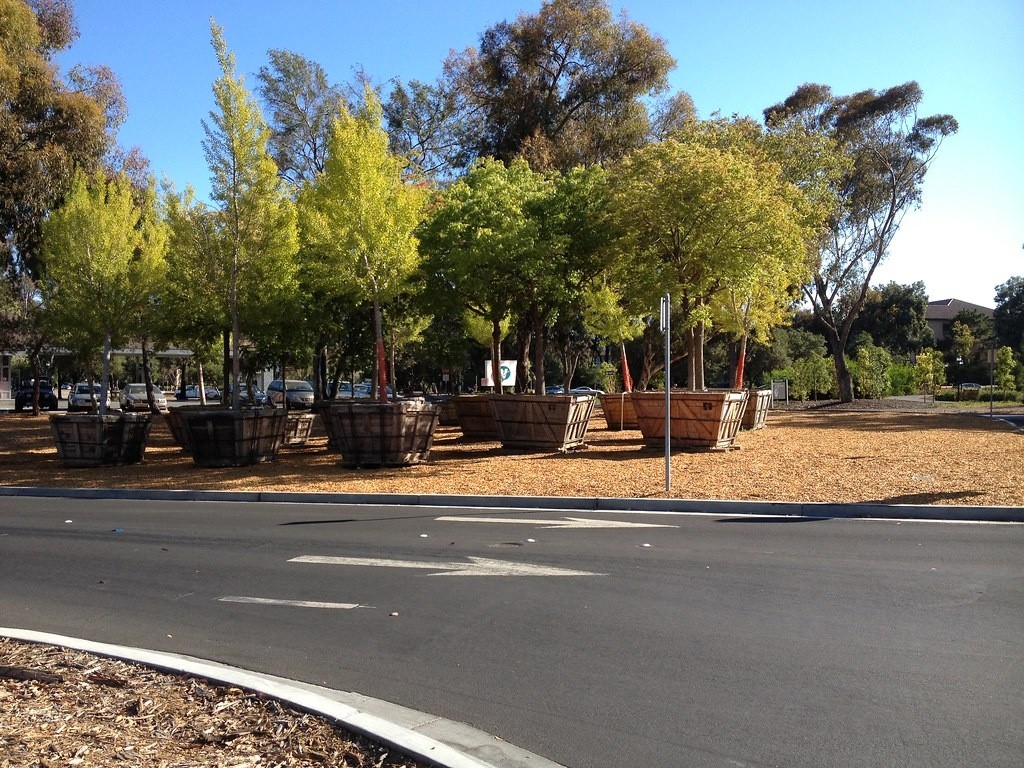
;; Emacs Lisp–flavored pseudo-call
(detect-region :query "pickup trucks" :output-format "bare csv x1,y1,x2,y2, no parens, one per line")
173,384,220,399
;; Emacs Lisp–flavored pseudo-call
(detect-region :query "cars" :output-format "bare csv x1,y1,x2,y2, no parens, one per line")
68,383,111,412
546,385,565,394
963,383,983,389
570,386,605,397
220,384,268,407
326,380,404,400
118,383,167,410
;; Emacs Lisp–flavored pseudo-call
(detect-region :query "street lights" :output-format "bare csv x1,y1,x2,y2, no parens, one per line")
956,354,961,394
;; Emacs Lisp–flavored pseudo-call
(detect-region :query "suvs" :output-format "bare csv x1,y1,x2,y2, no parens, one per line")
266,379,315,409
14,376,59,412
